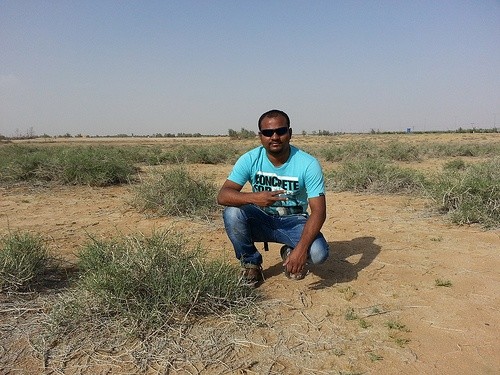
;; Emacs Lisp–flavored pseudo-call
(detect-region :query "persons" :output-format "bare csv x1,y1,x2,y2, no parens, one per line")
217,110,328,288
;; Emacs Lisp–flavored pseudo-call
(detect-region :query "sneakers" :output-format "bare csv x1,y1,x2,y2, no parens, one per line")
242,263,266,288
280,246,309,279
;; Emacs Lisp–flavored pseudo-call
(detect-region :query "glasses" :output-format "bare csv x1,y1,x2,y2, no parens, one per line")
260,127,288,136
